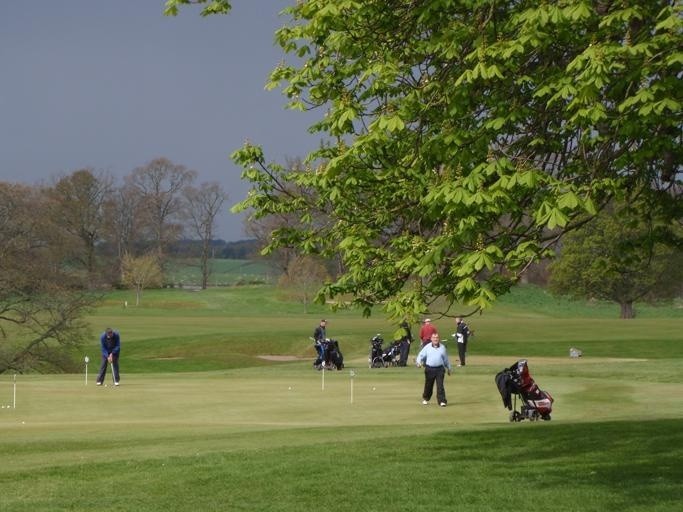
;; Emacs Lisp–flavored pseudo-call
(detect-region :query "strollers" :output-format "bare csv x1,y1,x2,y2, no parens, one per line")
370,338,402,368
316,338,344,371
494,358,554,423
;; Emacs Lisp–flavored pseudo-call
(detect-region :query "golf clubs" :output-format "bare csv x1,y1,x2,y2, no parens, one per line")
110,362,120,386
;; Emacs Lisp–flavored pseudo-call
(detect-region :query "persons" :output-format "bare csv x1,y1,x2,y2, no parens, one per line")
311,317,329,370
452,316,471,368
418,316,438,348
95,327,121,388
413,332,452,409
397,314,413,369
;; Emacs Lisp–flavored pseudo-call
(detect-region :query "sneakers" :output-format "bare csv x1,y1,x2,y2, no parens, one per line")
439,402,446,407
114,381,119,386
422,399,427,405
96,381,103,385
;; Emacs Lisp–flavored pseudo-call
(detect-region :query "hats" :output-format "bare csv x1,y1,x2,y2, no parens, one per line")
105,327,113,339
424,319,431,322
321,319,327,321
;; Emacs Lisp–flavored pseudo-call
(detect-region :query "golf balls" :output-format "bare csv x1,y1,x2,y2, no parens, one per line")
288,387,291,390
22,420,25,423
373,387,376,390
2,406,4,408
7,405,10,409
104,384,108,387
